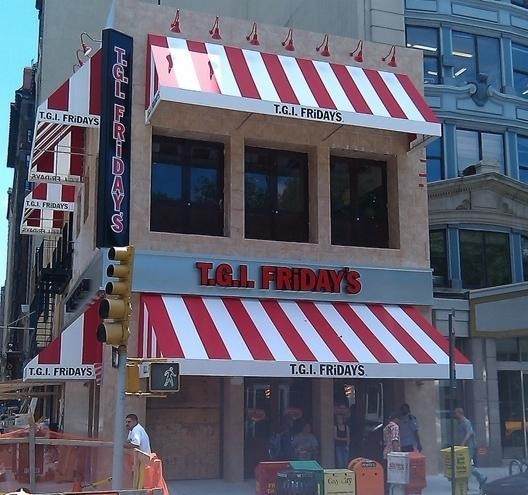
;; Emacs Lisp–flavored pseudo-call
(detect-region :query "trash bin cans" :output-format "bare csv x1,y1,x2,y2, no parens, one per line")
277,470,318,495
476,447,489,468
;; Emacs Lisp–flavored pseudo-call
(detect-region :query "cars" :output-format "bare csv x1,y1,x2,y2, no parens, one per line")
359,410,453,448
504,408,528,446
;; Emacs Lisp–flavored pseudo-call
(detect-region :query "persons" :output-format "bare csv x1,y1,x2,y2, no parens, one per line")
452,407,475,459
291,415,351,469
382,403,423,459
123,413,152,457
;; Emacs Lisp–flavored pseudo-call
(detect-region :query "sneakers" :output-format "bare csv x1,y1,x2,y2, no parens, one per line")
479,476,487,489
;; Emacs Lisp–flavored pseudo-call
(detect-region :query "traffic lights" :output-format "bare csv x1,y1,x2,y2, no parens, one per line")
96,245,135,345
148,362,181,398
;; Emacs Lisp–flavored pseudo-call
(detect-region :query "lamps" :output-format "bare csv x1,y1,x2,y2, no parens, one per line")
170,7,397,69
79,31,104,57
